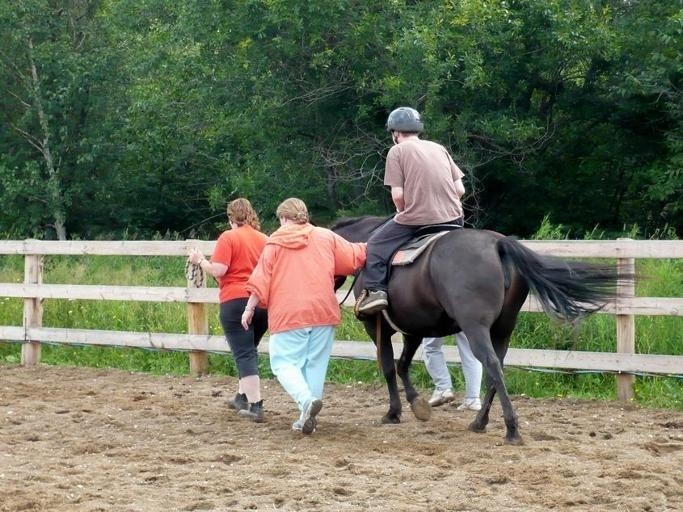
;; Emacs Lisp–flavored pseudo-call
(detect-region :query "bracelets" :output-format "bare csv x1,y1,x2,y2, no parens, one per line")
196,257,203,265
244,306,254,311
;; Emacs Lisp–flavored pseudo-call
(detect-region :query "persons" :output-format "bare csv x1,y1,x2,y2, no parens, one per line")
420,330,482,413
358,107,465,314
240,197,369,436
187,197,270,422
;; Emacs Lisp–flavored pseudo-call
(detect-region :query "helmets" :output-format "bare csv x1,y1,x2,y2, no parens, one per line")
386,107,424,135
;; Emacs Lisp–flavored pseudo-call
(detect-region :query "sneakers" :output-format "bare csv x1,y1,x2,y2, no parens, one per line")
291,397,322,434
227,392,249,411
457,397,481,411
237,400,265,423
357,289,388,315
427,388,454,407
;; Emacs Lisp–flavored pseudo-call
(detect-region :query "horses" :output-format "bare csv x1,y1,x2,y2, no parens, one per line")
307,214,652,446
